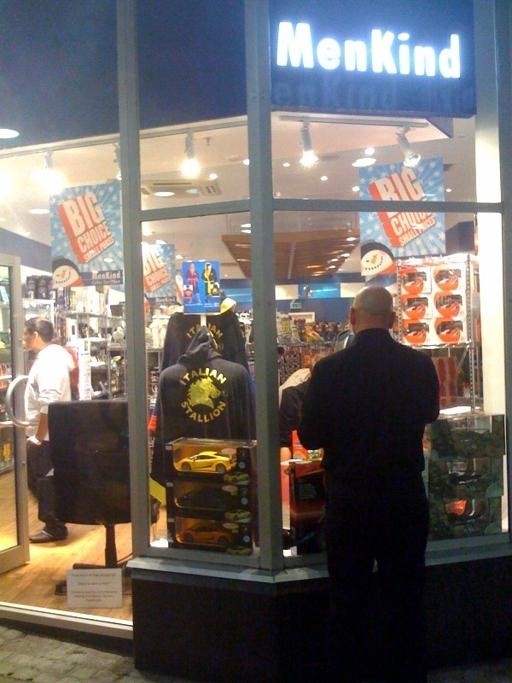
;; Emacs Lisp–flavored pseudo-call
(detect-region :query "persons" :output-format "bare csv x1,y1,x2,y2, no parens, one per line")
23,318,70,542
186,262,220,303
278,286,440,683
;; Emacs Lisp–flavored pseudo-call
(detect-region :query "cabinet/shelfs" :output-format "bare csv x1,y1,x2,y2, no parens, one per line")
3,275,165,472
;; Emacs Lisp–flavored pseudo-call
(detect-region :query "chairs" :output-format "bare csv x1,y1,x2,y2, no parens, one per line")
46,400,162,574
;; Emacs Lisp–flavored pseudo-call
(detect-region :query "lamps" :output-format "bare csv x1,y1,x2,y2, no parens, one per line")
1,126,21,140
295,121,422,171
181,131,201,180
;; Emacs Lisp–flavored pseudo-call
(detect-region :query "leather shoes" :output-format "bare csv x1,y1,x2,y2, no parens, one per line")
29,526,67,543
